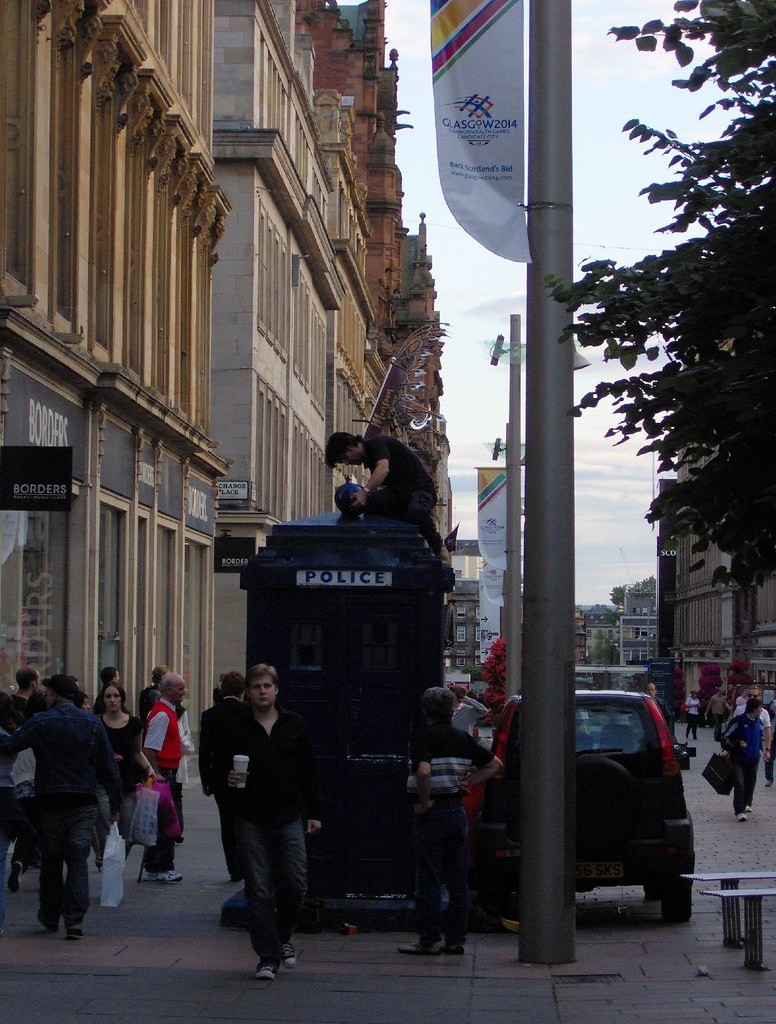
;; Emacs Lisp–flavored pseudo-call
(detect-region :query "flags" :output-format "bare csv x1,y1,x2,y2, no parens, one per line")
444,526,458,551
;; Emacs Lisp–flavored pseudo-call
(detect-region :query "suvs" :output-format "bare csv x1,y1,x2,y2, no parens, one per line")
463,688,696,925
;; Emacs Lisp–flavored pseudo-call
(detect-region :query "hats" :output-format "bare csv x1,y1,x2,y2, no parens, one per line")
43,673,77,699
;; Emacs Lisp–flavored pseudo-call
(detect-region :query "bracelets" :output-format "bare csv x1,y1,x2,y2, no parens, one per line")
363,488,369,494
766,748,771,751
146,766,151,770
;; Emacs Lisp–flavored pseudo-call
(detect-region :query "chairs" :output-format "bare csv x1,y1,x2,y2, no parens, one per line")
599,725,638,752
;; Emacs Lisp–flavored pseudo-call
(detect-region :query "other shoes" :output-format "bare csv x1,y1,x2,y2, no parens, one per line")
8,861,22,892
745,805,752,812
440,941,465,954
397,943,439,955
693,737,696,739
737,813,747,821
435,543,450,570
765,780,773,786
686,734,688,738
38,909,59,930
67,929,84,939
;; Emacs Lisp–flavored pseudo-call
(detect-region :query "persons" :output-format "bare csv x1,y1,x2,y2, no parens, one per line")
646,682,668,722
324,432,453,569
684,692,700,740
0,664,246,939
722,686,776,822
217,661,328,980
396,687,503,955
705,688,732,741
448,685,489,737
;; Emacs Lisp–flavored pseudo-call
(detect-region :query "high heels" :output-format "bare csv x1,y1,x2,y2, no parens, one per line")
95,858,103,872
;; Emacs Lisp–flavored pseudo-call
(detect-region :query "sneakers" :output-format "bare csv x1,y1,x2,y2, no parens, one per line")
279,942,296,968
255,962,278,979
142,870,183,881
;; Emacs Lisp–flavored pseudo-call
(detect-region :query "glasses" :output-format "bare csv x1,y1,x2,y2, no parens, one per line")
44,691,57,698
748,694,758,697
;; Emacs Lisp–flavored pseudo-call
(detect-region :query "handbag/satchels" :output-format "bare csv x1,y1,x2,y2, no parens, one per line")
685,702,690,711
129,778,160,849
135,778,181,838
100,822,124,906
702,753,735,795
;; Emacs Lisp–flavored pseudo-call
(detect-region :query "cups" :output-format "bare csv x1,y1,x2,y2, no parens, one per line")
233,755,249,788
